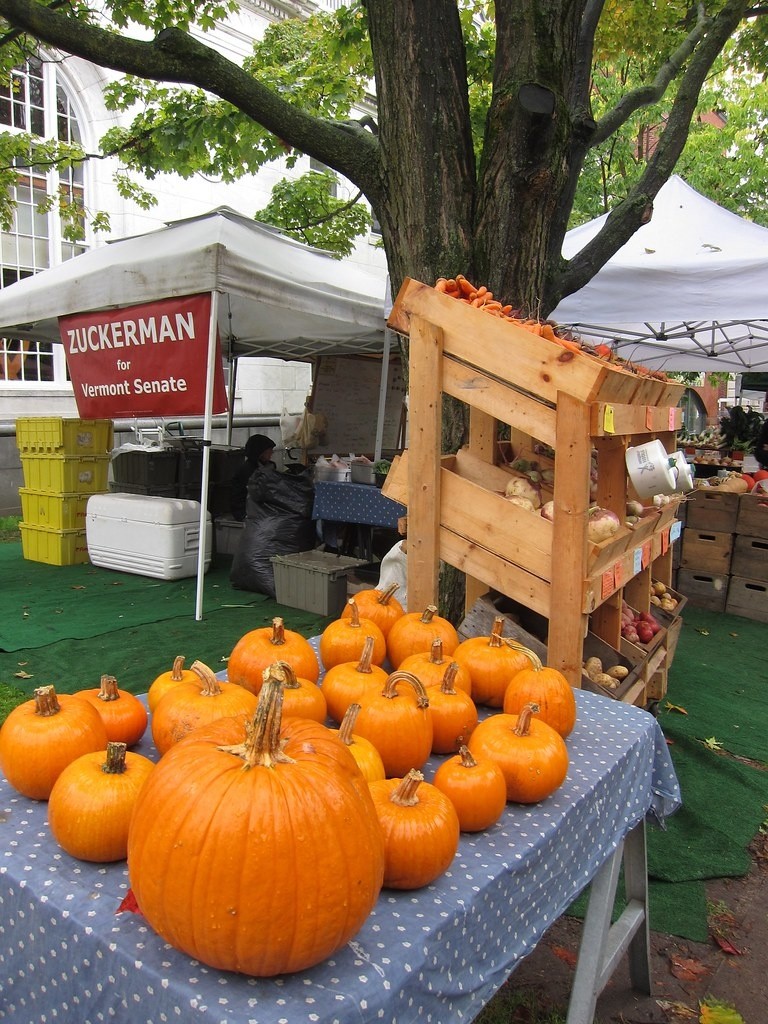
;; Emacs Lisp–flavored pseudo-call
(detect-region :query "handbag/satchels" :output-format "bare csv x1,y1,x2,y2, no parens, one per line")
304,409,319,449
279,408,302,449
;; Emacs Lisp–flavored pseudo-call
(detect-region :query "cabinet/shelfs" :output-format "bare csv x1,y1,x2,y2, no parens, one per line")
386,277,689,708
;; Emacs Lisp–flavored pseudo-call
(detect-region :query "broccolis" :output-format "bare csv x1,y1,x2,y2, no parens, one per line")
373,458,393,475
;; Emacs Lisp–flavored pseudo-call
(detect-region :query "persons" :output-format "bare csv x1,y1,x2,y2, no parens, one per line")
232,434,276,521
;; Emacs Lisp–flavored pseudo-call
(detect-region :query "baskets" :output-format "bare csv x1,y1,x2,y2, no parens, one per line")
373,472,387,488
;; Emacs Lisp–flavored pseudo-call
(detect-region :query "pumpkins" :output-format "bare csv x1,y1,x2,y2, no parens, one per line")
692,456,768,499
0,580,577,976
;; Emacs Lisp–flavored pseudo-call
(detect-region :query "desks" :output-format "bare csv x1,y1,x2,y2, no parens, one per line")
0,637,685,1024
312,483,407,563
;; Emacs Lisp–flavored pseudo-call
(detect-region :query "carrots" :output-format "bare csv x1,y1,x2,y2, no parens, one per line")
435,274,582,357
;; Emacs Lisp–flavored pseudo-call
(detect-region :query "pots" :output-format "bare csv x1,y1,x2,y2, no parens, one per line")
319,468,351,482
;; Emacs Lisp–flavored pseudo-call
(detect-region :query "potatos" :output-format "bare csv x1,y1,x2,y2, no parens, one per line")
500,581,680,693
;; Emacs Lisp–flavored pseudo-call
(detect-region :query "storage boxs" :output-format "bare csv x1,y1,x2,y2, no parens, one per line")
270,550,382,617
669,443,768,622
16,416,247,581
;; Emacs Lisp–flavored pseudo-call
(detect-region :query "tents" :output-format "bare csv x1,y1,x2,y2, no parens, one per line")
375,174,768,464
735,372,768,405
0,206,389,620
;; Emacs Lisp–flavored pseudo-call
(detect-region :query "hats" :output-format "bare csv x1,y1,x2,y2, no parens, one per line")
244,434,275,460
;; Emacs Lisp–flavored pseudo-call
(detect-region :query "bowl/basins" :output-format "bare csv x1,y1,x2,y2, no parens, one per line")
371,472,387,490
350,462,375,483
